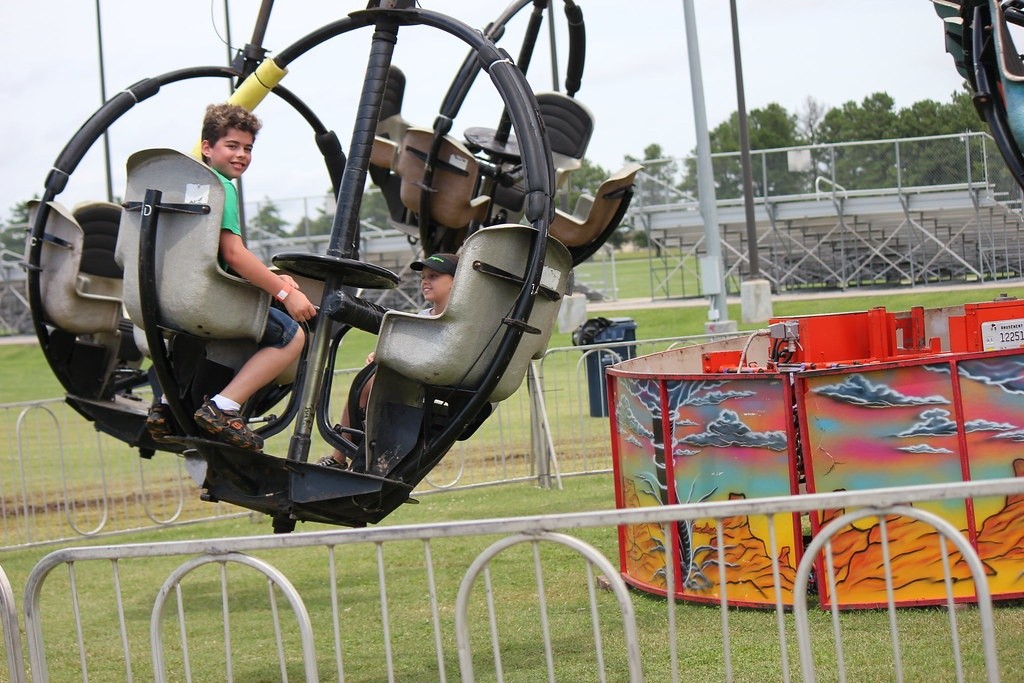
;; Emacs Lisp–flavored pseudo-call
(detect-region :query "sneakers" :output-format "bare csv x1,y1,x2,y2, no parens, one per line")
313,456,348,470
193,395,264,448
146,403,181,444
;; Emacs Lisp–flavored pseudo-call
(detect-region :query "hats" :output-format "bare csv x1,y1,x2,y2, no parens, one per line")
410,253,459,276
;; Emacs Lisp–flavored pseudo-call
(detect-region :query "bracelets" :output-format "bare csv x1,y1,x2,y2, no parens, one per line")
276,284,292,301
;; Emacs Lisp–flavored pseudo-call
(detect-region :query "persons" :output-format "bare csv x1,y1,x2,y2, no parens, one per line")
146,103,316,449
311,253,459,469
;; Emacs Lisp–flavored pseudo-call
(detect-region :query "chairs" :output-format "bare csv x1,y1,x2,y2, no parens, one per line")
115,148,312,421
368,65,644,248
364,225,574,441
65,201,145,362
22,195,123,401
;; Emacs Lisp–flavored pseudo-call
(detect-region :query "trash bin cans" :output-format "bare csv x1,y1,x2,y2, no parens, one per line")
583,317,638,418
